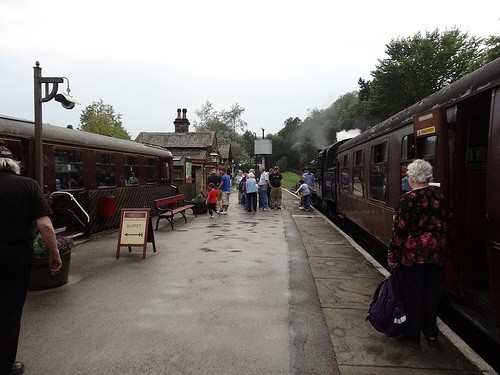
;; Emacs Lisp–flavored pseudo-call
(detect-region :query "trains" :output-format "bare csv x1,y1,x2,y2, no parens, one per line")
0,114,174,239
307,55,500,343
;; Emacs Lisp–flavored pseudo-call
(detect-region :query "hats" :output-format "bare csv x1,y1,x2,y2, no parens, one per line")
0,146,13,158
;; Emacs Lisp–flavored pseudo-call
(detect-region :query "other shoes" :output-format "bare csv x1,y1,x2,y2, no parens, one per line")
271,205,274,209
217,210,220,213
424,332,437,344
305,208,311,211
0,362,25,375
299,206,305,210
220,212,226,214
263,207,268,211
277,207,281,209
395,332,420,347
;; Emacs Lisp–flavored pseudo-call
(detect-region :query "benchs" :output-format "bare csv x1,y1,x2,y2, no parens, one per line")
152,193,198,232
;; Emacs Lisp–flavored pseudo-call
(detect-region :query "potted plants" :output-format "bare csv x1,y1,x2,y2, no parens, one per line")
26,249,72,292
193,192,208,215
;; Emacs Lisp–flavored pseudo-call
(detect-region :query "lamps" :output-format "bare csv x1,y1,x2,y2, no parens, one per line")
210,146,224,167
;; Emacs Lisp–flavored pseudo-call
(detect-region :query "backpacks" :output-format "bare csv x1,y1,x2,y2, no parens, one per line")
364,268,409,337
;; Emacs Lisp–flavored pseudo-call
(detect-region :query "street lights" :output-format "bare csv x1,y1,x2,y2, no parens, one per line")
33,59,80,188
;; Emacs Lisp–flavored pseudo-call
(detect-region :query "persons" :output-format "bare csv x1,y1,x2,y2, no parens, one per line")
206,183,220,218
295,168,317,211
217,168,231,214
401,166,412,192
0,146,62,375
60,172,139,189
387,159,447,349
207,169,221,209
235,166,283,211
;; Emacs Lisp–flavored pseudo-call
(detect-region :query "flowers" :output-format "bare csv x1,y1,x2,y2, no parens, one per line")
29,232,72,258
